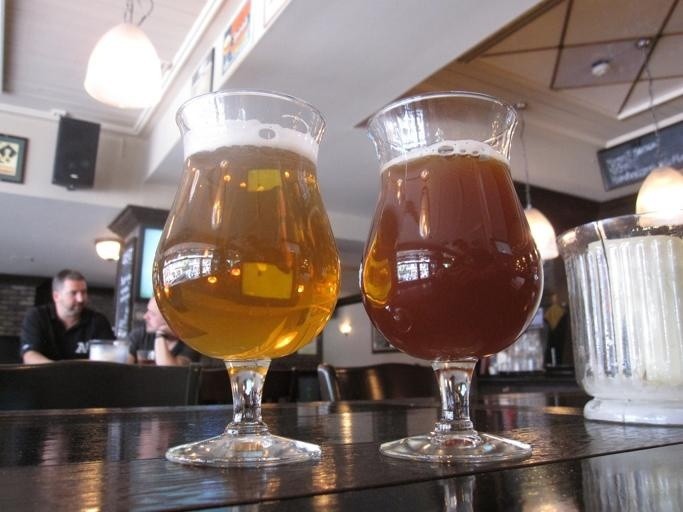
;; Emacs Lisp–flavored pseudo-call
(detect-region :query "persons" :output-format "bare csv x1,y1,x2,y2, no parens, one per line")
126,296,201,368
18,269,115,364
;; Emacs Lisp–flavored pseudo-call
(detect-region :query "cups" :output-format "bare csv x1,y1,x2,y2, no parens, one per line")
137,350,156,368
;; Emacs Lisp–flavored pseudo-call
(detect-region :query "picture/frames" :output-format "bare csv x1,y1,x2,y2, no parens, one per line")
370,322,400,354
0,132,29,183
135,224,166,304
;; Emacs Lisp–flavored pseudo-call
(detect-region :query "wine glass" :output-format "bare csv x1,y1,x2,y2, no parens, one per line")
151,91,340,466
358,89,545,466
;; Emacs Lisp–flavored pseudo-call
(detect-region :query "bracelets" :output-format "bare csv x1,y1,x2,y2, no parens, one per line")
154,334,168,338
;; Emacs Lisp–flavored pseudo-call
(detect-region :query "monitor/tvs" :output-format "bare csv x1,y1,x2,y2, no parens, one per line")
136,225,163,305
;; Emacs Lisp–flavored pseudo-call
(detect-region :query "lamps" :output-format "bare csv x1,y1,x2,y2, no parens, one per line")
633,34,683,231
512,101,559,262
83,0,165,109
93,238,122,262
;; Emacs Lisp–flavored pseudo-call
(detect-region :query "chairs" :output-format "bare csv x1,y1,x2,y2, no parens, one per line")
287,363,320,402
0,359,206,409
316,361,480,402
199,366,287,404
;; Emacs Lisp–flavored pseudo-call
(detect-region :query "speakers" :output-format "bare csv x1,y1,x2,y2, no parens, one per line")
52,116,100,189
